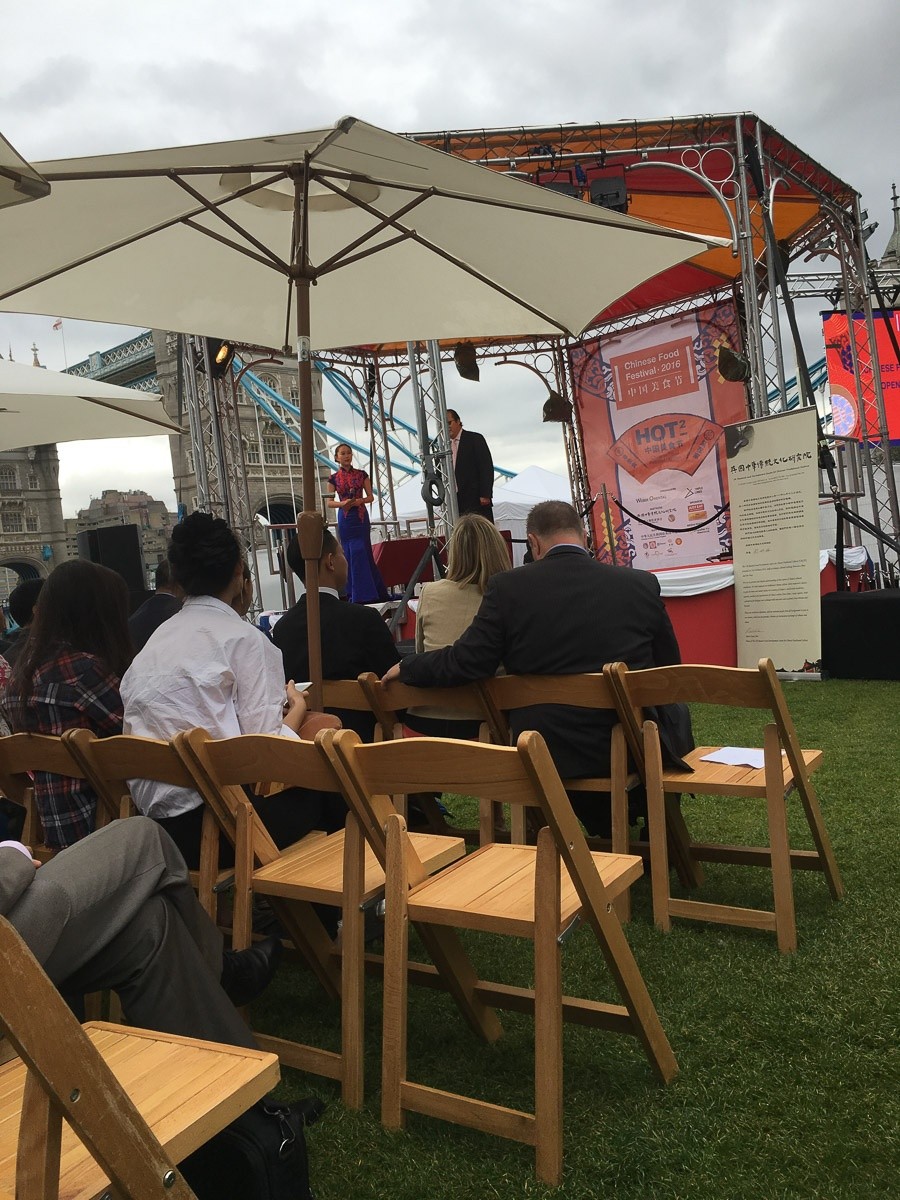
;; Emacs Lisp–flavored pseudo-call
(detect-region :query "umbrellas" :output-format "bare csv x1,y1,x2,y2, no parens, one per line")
1,116,737,721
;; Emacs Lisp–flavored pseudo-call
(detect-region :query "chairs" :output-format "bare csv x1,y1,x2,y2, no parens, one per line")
0,657,845,1200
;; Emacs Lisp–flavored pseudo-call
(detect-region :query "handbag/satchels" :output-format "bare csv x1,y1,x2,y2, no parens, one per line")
176,1098,310,1200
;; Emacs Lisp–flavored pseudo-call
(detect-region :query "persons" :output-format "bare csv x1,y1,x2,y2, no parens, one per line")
447,410,495,529
0,498,696,948
328,444,385,603
1,815,322,1128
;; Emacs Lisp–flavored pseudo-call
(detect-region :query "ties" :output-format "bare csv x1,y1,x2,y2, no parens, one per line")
451,439,458,464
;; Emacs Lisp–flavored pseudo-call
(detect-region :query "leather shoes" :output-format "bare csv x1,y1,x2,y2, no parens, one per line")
220,936,284,1007
281,1095,326,1137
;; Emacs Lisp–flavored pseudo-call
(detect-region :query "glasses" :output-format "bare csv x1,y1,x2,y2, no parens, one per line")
249,569,256,582
447,418,458,424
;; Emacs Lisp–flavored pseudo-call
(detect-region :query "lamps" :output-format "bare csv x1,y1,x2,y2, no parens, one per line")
541,391,573,425
453,338,483,382
719,345,752,382
186,334,237,380
535,160,632,216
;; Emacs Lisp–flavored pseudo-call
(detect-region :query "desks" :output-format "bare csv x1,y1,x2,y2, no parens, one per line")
370,529,512,597
820,589,900,681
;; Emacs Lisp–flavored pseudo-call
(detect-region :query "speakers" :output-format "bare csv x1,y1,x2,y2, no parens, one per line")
544,181,583,200
129,589,158,616
77,523,147,591
590,176,629,215
